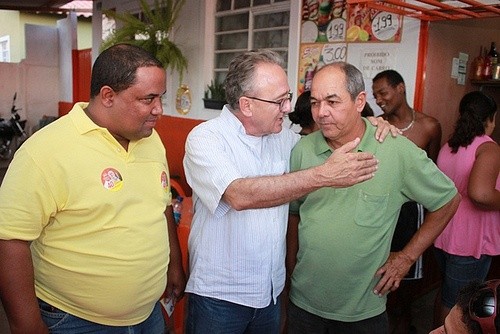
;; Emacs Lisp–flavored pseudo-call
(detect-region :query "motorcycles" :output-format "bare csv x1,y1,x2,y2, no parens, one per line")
0,92,29,160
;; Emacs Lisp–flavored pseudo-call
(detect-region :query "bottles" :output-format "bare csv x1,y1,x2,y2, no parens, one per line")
173,197,184,225
488,41,499,79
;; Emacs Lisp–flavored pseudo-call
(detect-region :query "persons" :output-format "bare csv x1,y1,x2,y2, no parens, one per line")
290,69,443,334
436,90,500,322
182,50,402,334
0,43,185,334
428,278,500,334
285,62,462,334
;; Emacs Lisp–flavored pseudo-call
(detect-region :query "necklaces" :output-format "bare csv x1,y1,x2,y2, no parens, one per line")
397,109,417,131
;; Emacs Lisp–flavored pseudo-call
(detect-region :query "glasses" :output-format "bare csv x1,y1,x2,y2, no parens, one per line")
468,279,500,334
237,93,293,108
